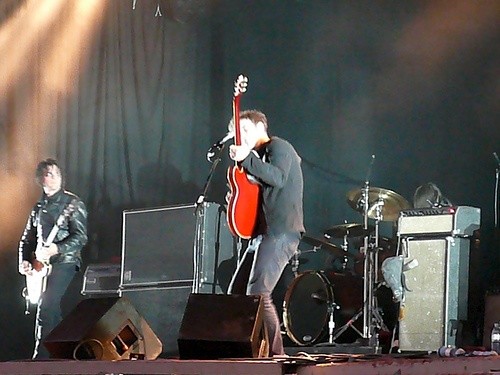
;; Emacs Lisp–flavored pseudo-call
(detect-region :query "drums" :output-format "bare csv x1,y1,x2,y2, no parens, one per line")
281,267,373,347
354,235,397,280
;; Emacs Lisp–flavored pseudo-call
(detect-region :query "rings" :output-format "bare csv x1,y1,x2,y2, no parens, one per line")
231,156,234,159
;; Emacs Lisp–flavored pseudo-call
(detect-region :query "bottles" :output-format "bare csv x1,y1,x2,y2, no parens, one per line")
490,323,500,355
437,346,464,357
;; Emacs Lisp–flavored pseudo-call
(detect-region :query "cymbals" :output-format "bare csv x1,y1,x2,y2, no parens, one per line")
324,221,377,237
344,184,413,223
304,234,354,260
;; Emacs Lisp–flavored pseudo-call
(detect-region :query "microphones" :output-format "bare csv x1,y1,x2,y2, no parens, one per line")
208,132,234,154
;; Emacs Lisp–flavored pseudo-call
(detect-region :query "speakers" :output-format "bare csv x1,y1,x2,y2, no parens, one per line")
122,202,238,287
118,285,246,359
177,293,269,361
44,298,145,361
399,239,471,354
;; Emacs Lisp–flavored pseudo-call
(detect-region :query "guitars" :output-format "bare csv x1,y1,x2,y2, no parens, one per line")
221,71,266,239
25,206,74,305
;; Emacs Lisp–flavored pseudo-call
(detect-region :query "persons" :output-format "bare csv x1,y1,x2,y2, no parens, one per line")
18,159,87,359
228,109,304,355
